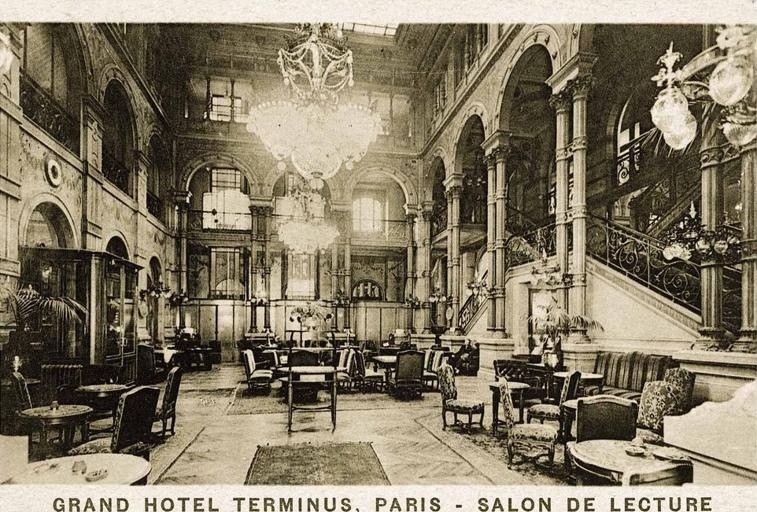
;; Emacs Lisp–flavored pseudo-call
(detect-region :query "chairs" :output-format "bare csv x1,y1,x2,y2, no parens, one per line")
231,327,484,438
475,343,710,487
0,324,222,486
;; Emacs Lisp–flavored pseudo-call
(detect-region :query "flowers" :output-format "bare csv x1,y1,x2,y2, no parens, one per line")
285,300,336,328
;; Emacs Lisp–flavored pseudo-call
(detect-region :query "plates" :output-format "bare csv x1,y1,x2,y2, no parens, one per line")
651,449,673,460
624,447,646,456
85,468,107,480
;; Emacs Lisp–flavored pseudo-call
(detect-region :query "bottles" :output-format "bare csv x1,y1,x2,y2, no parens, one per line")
72,459,86,475
50,401,59,412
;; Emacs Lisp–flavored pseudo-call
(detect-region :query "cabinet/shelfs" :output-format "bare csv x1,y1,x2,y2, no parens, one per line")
16,242,146,392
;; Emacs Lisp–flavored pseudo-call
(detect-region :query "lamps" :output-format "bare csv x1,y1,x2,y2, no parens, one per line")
274,181,340,257
645,23,757,152
653,197,744,273
239,23,386,192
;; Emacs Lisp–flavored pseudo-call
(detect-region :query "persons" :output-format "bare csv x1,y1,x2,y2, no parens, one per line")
448,339,479,376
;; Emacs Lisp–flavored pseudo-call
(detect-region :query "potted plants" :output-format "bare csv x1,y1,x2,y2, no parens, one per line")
514,294,608,376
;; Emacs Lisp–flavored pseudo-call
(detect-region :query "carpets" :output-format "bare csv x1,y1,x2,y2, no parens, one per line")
241,437,392,485
222,365,519,417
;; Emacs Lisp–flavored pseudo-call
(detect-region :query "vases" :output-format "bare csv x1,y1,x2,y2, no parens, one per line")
299,313,328,349
